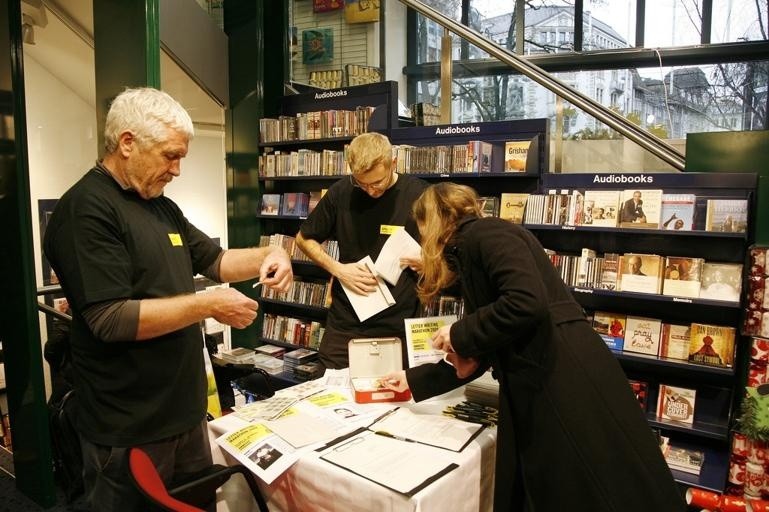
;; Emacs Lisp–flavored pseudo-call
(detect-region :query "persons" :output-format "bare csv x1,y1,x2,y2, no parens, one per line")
42,87,295,512
376,182,688,512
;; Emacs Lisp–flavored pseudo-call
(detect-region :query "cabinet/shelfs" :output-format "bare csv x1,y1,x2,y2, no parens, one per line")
255,71,759,495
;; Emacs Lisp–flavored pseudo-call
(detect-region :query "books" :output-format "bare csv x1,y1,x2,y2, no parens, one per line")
391,140,530,175
419,293,465,325
477,189,748,474
211,105,377,382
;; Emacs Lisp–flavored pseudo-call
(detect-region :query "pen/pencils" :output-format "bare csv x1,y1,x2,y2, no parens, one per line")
443,400,500,427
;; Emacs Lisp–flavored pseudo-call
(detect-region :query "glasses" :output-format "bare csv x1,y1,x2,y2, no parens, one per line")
350,170,391,189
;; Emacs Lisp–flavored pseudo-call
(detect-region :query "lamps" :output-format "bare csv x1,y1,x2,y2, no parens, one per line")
19,0,48,45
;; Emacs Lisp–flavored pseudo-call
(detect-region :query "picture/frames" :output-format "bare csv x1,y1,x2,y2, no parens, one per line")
38,199,70,340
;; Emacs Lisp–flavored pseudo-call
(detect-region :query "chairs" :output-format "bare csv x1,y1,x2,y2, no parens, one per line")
232,368,273,403
123,445,269,512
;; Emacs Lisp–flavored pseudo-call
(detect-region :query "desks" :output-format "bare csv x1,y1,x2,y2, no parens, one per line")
213,347,310,392
207,347,500,512
